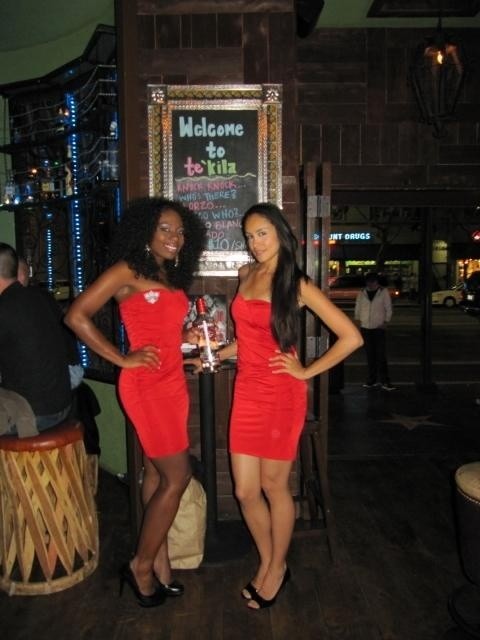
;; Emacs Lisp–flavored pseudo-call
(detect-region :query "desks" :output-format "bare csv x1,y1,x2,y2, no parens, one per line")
180,354,256,565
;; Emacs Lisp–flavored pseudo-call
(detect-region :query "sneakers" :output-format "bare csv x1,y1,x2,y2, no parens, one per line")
361,379,381,389
380,380,398,392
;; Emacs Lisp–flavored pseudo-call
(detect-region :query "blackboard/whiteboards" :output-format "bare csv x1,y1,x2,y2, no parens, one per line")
161,98,269,263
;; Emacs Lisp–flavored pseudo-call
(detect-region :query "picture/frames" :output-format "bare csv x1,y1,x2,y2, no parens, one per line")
144,80,282,280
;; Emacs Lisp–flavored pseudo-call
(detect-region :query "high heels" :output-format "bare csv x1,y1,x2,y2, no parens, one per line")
152,571,186,598
118,563,165,609
241,563,292,611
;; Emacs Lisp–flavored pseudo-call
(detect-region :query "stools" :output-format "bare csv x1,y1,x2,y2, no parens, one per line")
283,415,346,569
448,463,480,639
1,418,102,596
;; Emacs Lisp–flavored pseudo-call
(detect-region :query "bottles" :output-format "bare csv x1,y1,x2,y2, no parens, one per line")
191,295,224,376
2,138,120,204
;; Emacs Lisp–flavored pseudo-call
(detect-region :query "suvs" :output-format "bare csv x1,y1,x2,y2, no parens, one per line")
460,270,480,319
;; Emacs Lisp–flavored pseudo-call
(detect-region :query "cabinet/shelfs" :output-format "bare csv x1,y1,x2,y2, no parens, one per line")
0,96,119,216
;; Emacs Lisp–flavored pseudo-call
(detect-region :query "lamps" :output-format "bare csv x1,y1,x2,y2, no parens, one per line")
406,1,463,141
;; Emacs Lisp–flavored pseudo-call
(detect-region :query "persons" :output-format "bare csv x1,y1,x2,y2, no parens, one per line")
408,271,417,300
61,197,210,609
354,276,396,391
393,273,401,301
17,259,85,392
1,242,73,436
182,201,366,611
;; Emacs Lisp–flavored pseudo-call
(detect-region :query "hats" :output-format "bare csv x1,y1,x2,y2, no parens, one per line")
364,272,378,283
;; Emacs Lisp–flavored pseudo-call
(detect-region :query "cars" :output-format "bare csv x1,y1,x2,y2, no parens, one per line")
430,280,463,308
327,272,400,303
